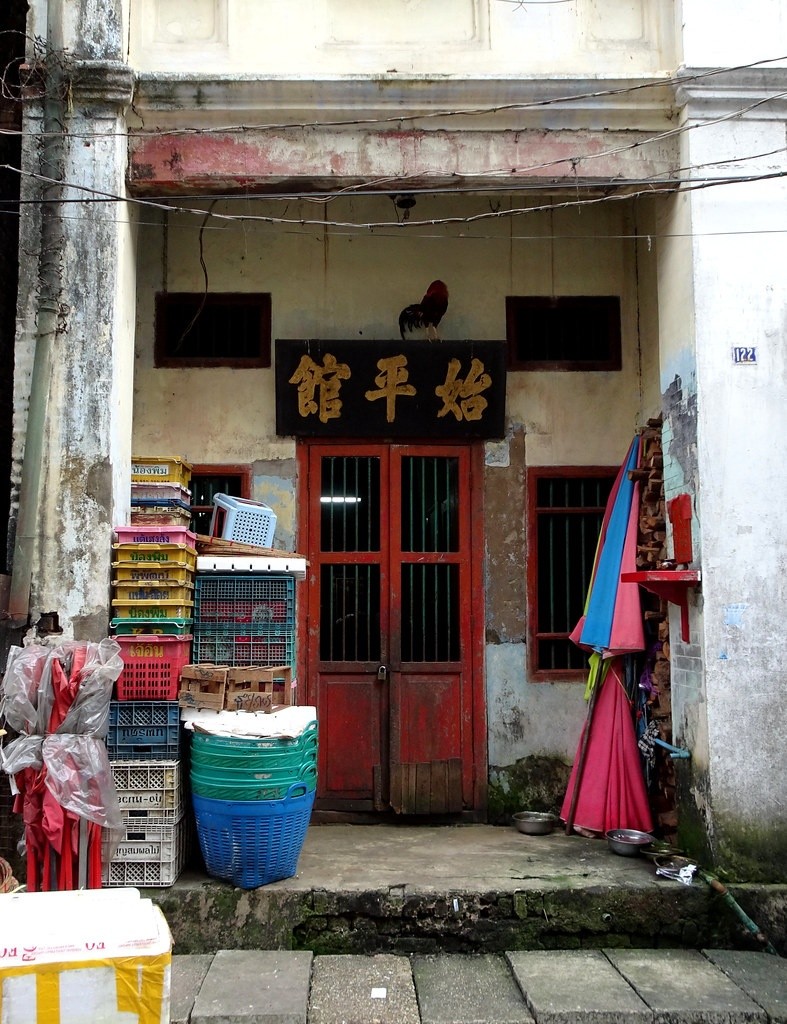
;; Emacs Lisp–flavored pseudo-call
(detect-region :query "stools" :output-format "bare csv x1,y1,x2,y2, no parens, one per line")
209,492,277,549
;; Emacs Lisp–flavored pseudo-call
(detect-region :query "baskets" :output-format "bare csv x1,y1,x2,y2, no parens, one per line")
95,634,193,888
188,715,320,888
193,574,300,681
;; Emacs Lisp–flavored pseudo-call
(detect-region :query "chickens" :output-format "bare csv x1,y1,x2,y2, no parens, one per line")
399,280,449,343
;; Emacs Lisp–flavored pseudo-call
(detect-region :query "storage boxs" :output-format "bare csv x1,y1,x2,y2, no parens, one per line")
109,527,198,700
131,455,192,529
179,663,290,713
194,575,297,681
0,887,175,1023
101,760,188,888
109,702,189,760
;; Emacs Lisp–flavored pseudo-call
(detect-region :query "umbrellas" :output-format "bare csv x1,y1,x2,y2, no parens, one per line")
564,435,654,837
